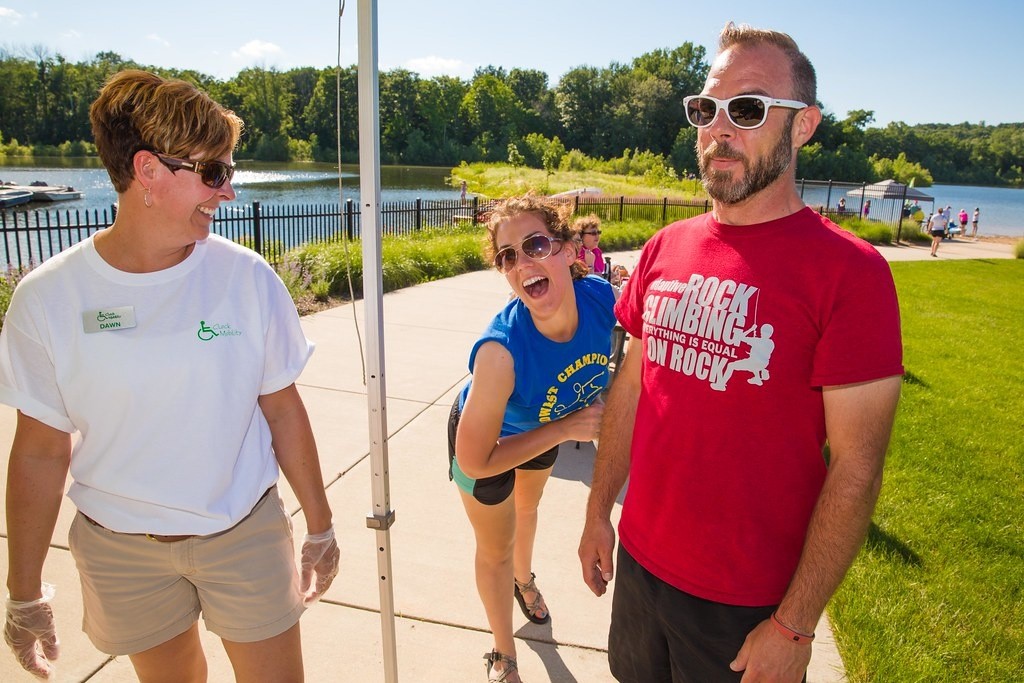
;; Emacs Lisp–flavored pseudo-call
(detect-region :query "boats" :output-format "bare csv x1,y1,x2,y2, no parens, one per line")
0,180,84,210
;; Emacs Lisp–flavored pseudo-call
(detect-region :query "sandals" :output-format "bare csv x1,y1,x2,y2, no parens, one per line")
514,573,549,624
483,648,524,683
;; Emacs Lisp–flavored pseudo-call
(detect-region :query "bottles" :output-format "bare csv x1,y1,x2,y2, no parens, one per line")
588,265,592,274
619,277,630,295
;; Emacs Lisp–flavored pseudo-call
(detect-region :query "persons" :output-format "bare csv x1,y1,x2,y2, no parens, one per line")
959,209,969,237
577,212,605,276
927,205,958,258
447,188,622,683
0,73,339,683
971,208,980,237
904,199,925,226
864,201,871,217
837,196,846,213
578,21,904,683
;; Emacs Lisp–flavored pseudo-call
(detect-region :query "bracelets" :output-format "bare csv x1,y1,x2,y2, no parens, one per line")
587,265,594,268
770,611,815,644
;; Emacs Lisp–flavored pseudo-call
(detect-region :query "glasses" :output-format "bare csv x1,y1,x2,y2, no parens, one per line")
494,236,563,273
683,94,808,130
156,152,238,188
582,230,602,237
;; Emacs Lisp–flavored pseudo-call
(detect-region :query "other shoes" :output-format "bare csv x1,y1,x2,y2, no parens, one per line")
931,253,937,257
972,237,978,242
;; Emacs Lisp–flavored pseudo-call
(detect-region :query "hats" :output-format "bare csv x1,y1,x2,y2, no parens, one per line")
840,198,845,202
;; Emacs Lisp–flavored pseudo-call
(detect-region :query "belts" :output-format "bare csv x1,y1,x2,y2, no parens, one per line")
78,488,273,542
